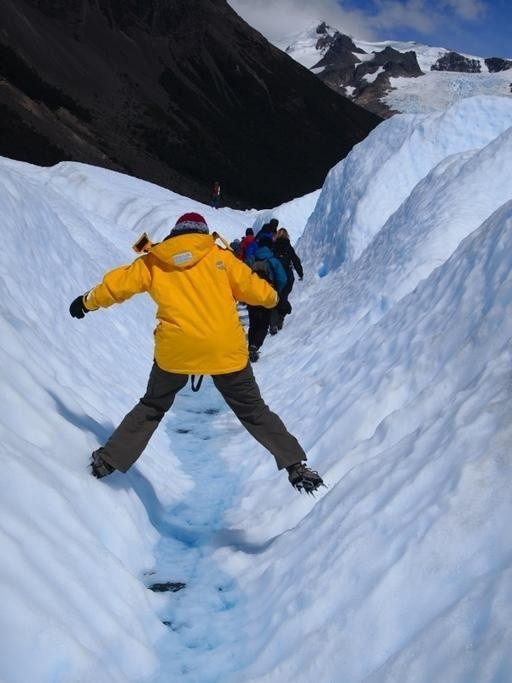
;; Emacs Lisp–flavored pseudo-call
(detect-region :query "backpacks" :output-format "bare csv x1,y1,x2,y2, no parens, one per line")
249,254,277,290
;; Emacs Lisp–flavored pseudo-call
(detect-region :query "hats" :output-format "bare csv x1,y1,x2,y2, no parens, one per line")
170,212,211,232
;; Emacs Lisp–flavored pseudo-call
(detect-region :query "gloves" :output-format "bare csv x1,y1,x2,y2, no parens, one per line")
69,295,88,318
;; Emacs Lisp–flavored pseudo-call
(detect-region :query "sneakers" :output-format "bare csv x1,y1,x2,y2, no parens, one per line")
92,446,114,476
288,464,322,492
248,345,259,363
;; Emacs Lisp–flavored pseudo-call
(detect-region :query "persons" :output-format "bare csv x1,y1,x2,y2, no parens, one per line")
67,212,324,493
225,220,302,364
210,180,223,209
